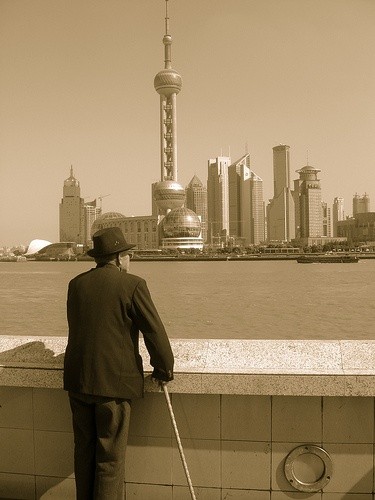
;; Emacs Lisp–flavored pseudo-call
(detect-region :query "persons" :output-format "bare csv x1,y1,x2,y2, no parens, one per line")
63,227,174,500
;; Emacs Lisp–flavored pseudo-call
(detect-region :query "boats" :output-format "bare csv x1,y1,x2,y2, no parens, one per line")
297,250,359,263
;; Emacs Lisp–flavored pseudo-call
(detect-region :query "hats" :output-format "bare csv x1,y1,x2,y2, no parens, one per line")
87,226,136,258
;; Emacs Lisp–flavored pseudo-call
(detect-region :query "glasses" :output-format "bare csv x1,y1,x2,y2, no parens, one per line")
126,250,135,258
